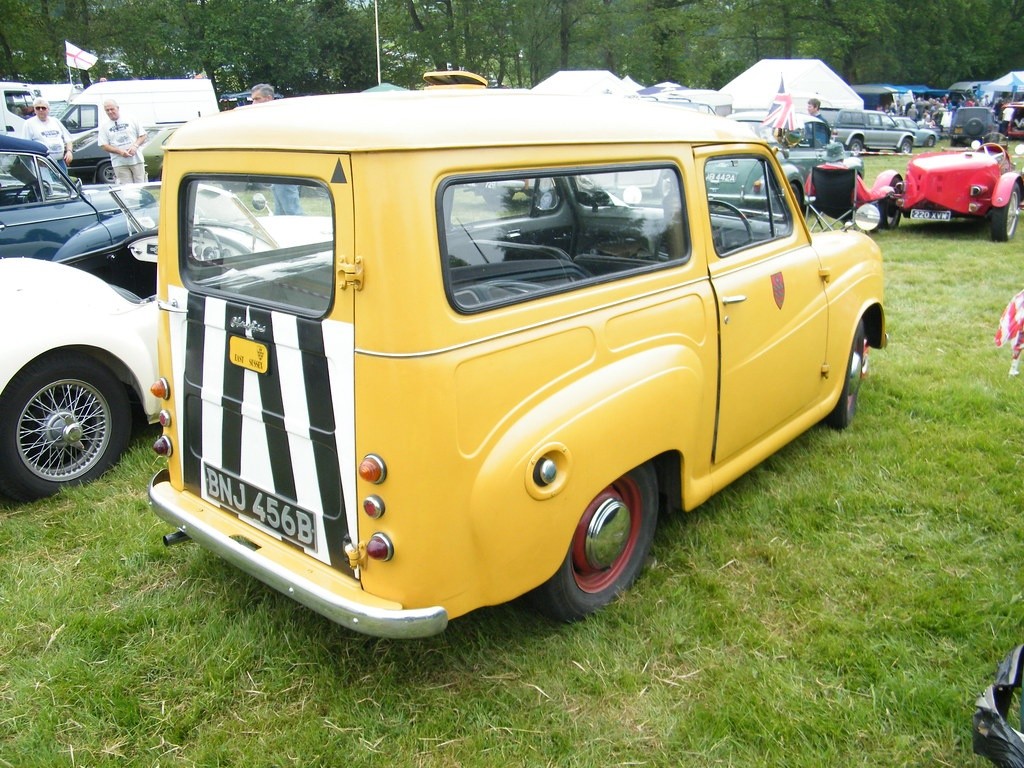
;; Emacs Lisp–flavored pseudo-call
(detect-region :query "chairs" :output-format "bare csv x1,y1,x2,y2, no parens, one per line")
574,254,664,275
466,238,573,262
805,165,895,232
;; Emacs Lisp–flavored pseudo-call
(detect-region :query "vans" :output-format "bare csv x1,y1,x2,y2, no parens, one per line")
0,82,45,142
56,77,221,184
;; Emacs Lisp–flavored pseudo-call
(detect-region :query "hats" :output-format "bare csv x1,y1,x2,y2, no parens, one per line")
822,142,850,162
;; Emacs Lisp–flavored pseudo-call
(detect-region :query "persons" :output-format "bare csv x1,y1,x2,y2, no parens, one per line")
807,98,831,146
805,142,895,232
21,97,72,174
251,83,303,216
99,78,107,82
97,99,147,184
876,89,1024,138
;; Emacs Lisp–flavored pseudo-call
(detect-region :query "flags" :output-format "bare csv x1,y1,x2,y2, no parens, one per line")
65,41,98,70
761,78,795,131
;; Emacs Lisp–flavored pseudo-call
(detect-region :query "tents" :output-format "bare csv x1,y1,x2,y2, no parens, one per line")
358,59,1023,112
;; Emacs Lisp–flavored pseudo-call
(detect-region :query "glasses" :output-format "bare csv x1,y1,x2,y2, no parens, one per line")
35,107,47,110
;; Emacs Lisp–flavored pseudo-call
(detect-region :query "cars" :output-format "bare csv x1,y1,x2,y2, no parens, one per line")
573,111,807,223
871,133,1024,242
147,71,890,638
0,125,335,503
891,117,939,148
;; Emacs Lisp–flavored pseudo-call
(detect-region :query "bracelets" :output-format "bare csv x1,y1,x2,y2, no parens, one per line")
133,143,139,148
66,150,73,153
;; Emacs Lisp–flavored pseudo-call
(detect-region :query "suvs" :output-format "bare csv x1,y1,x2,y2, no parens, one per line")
827,107,915,157
949,106,1003,147
